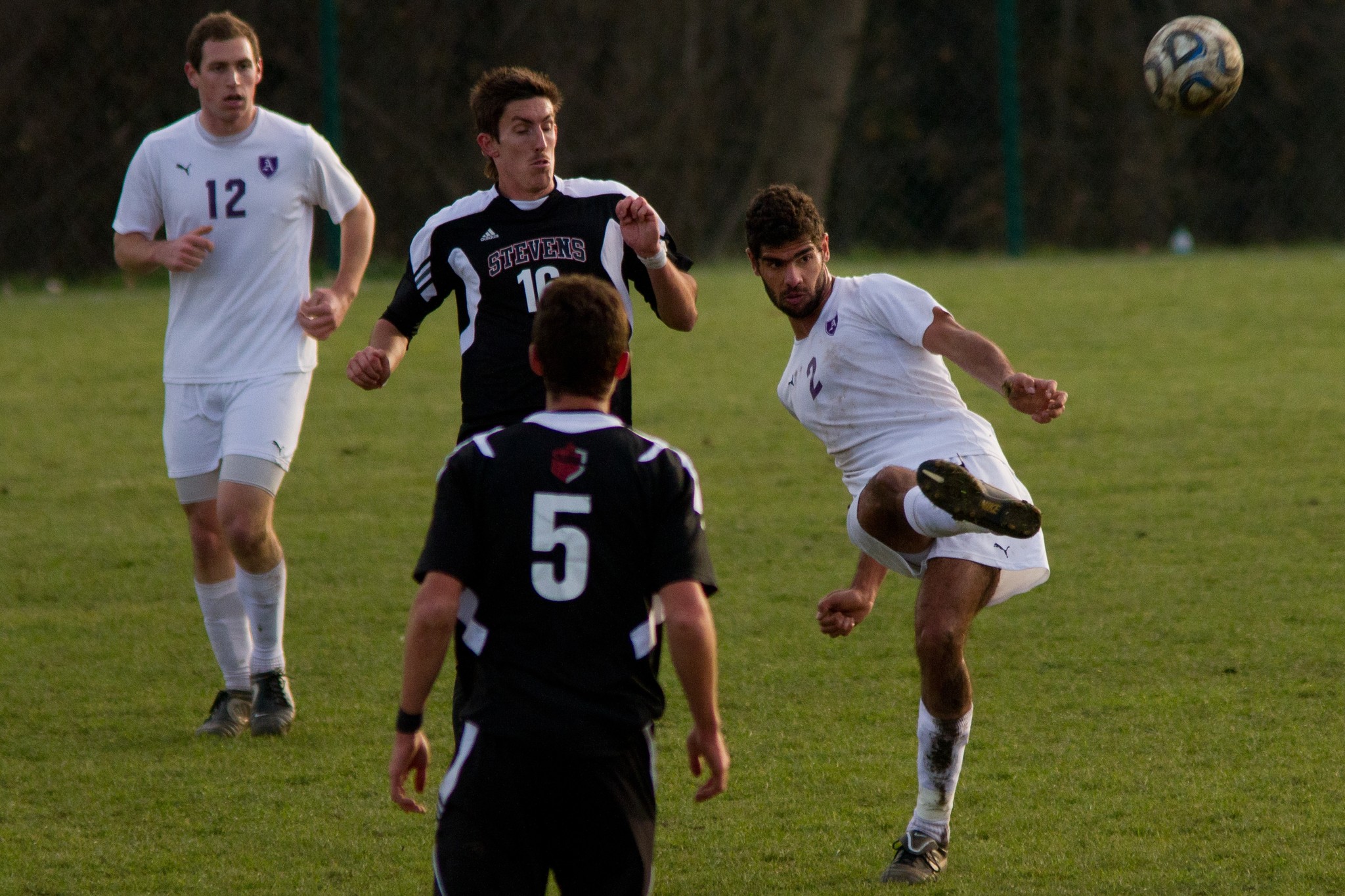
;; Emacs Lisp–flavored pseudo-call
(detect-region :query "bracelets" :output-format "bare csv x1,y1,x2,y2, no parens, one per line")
395,707,424,735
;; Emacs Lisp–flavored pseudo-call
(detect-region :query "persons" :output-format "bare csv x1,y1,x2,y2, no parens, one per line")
346,66,700,444
111,10,376,736
740,183,1070,885
386,275,730,895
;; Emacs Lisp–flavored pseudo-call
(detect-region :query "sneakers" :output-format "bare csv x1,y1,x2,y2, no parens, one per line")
196,689,256,741
883,830,954,884
916,459,1043,539
248,666,296,737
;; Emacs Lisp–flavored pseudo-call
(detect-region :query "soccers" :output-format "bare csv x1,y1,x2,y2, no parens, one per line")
1144,16,1244,119
